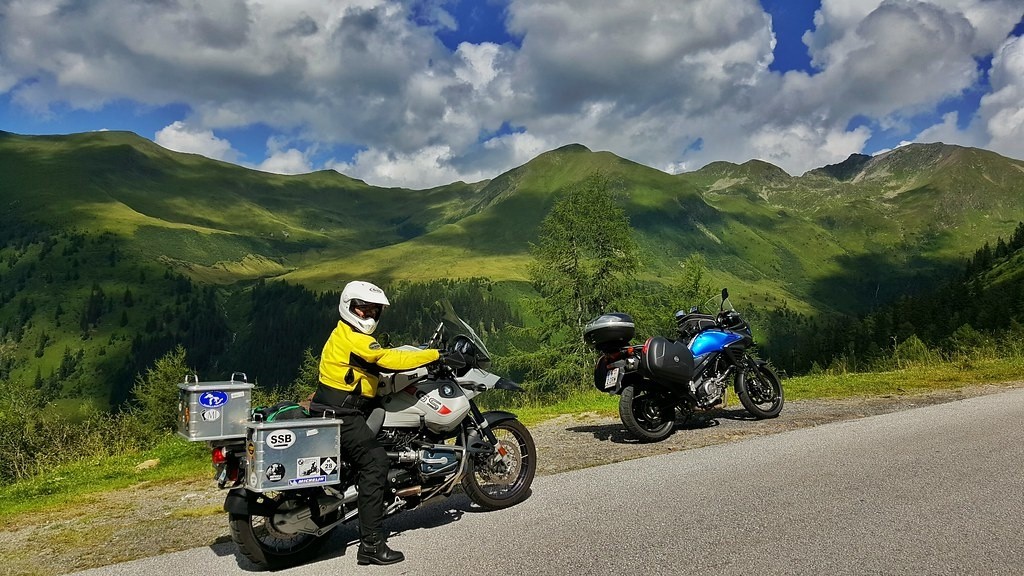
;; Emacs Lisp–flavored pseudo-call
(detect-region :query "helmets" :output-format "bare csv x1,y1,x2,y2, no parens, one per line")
339,280,392,336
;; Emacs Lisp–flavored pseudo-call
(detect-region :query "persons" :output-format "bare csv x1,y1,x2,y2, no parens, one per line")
308,280,449,565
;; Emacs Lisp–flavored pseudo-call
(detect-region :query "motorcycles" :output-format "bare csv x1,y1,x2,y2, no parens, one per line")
176,295,538,569
584,288,785,444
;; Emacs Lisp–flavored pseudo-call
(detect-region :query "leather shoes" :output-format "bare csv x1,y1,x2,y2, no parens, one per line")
355,542,405,566
